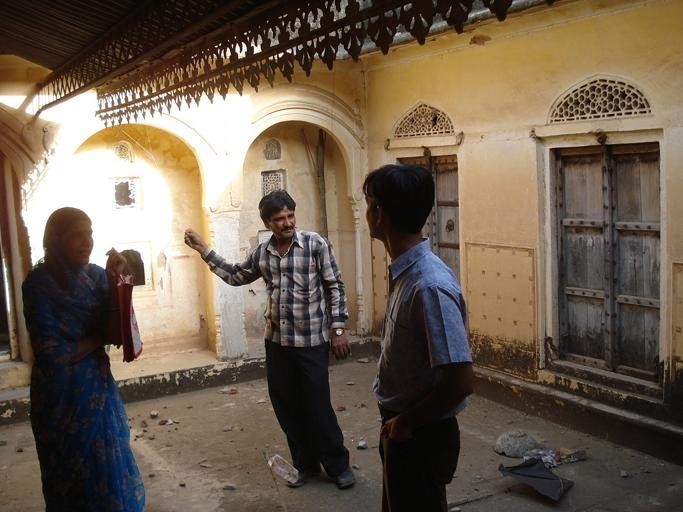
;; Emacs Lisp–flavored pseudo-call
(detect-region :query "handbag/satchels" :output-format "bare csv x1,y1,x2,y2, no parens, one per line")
114,268,143,364
96,282,124,347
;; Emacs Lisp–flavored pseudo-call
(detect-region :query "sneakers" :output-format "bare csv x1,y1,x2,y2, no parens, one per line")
332,463,355,489
284,464,321,488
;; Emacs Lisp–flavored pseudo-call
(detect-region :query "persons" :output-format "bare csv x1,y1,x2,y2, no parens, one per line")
19,206,146,512
360,163,474,511
183,188,358,490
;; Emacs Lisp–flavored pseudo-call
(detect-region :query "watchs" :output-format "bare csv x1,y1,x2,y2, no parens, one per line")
331,327,345,336
